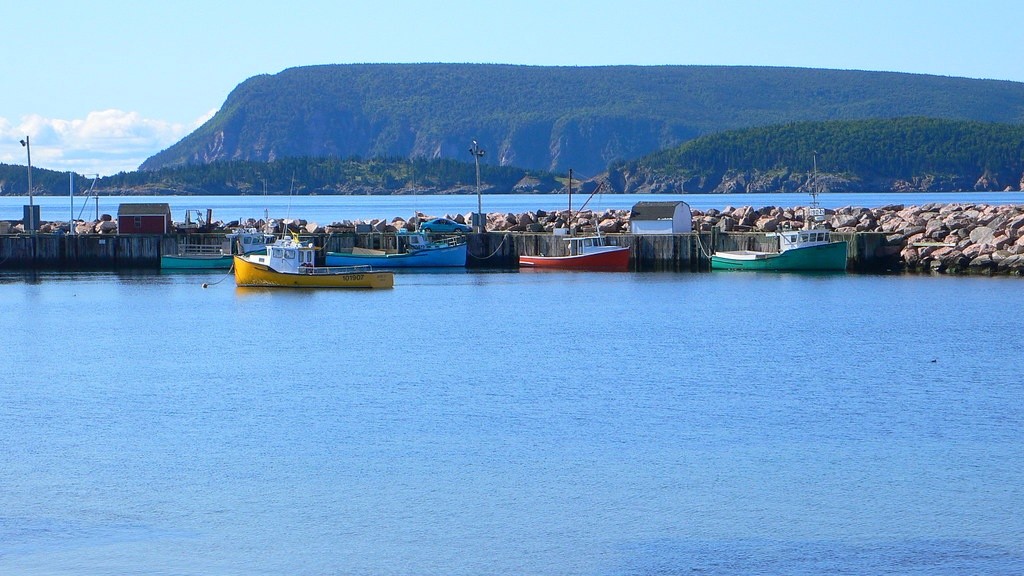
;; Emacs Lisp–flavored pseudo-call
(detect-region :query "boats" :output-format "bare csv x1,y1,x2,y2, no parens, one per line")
232,237,394,290
711,229,848,273
161,231,281,269
519,234,631,272
325,229,466,268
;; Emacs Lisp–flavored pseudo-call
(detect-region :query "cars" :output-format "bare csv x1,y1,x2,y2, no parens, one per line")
420,218,473,235
